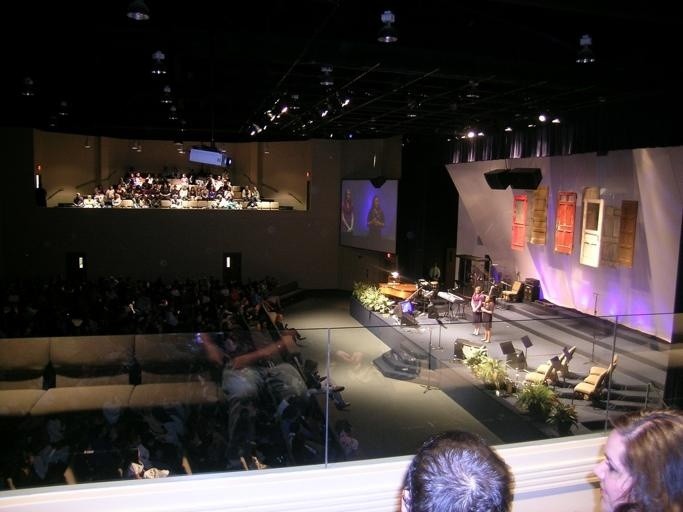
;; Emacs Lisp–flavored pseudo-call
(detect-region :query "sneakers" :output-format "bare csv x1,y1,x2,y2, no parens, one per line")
335,386,344,392
345,402,350,406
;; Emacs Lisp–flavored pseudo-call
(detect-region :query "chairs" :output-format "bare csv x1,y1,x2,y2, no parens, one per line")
72,168,281,209
523,345,619,404
502,282,524,303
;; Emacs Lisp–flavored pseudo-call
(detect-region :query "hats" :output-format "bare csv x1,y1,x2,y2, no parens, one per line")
143,468,169,479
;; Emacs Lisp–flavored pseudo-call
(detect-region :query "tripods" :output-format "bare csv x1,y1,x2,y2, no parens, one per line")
517,346,530,373
584,296,600,365
421,330,441,393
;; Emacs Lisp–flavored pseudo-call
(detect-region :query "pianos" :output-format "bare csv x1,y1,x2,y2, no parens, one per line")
380,283,417,299
437,292,464,303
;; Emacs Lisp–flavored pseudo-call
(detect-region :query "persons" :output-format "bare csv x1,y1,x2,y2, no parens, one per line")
481,295,495,343
367,196,385,232
428,261,441,280
398,428,513,512
73,167,263,210
341,188,353,233
592,410,682,511
470,285,484,335
1,266,357,485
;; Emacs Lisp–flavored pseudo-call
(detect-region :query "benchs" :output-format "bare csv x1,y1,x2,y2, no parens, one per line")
0,278,351,490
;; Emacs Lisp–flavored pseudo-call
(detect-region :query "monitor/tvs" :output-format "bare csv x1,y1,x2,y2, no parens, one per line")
398,300,413,316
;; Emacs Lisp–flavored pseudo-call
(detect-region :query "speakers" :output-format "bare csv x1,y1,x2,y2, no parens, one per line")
508,169,543,189
484,169,511,189
401,313,418,325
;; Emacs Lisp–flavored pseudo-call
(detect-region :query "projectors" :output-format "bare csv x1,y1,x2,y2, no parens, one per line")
189,146,232,168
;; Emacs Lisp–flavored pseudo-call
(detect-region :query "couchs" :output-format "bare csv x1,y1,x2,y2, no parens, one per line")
0,326,228,422
